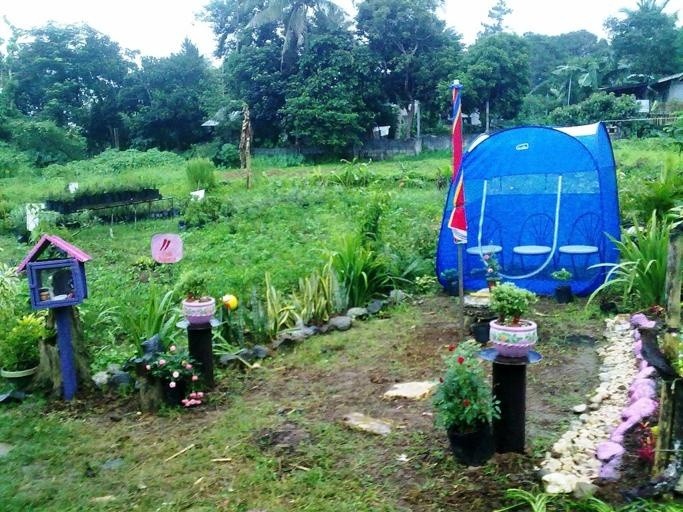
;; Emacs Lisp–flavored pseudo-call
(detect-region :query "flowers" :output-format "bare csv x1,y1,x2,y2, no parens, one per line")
439,337,499,428
144,343,203,407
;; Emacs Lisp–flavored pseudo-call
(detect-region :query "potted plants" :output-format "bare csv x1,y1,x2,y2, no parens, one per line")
485,281,540,358
173,271,216,325
183,158,212,201
48,180,162,212
0,315,45,391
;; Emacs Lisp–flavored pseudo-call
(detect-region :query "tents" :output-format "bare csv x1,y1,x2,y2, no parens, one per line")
431,118,623,304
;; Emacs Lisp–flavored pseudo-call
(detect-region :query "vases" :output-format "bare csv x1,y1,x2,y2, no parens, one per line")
440,422,492,466
139,374,185,412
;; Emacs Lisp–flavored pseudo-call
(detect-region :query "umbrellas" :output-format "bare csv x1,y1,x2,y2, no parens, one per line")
445,79,468,341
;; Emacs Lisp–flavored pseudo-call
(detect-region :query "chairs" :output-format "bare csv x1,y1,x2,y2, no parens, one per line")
466,213,604,281
